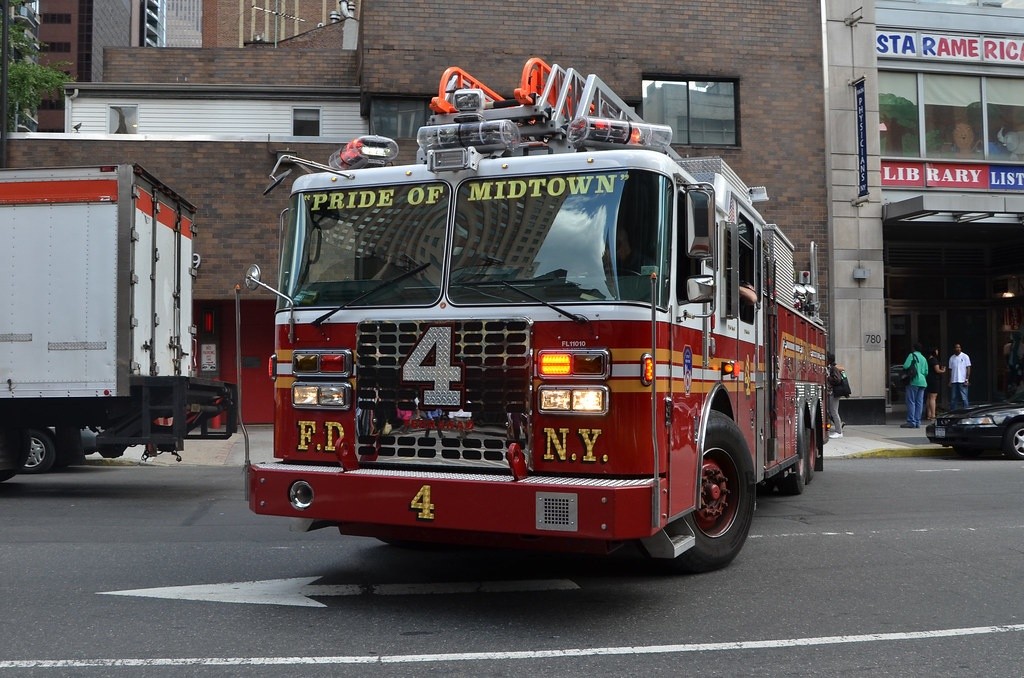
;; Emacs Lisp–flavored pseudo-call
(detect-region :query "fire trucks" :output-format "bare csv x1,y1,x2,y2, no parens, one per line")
0,163,240,483
244,56,830,575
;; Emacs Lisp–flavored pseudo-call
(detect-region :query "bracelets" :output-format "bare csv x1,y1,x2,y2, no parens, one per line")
965,379,969,383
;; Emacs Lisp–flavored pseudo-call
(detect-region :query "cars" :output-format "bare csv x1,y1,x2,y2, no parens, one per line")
926,391,1024,460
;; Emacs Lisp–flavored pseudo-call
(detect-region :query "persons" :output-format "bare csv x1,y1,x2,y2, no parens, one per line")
901,342,972,428
371,223,653,279
825,350,846,439
737,278,758,319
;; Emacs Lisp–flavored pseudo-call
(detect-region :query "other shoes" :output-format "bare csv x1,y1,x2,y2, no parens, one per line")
900,422,915,428
930,416,936,421
841,422,845,428
926,416,931,420
916,424,920,428
829,432,843,439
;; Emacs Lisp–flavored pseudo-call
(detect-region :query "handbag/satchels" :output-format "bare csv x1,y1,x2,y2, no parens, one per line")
832,377,851,398
900,352,919,383
